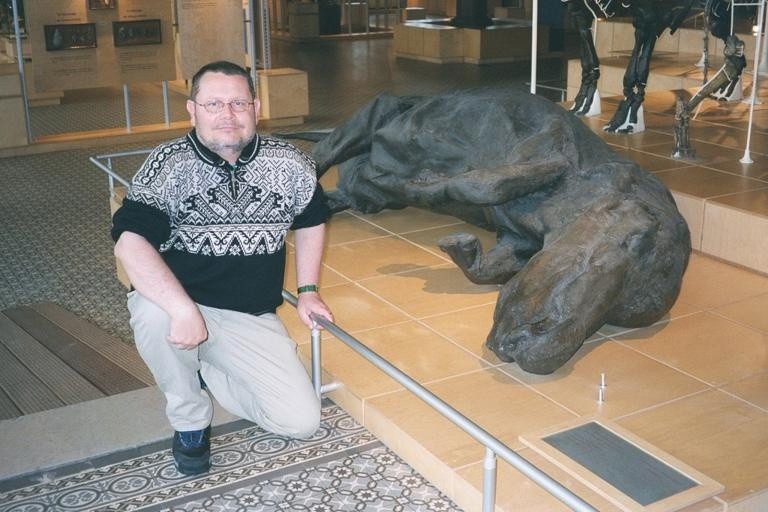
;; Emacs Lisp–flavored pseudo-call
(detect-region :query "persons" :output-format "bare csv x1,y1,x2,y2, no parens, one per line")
106,61,334,477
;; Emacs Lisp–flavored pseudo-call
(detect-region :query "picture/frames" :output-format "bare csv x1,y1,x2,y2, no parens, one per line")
111,19,162,47
88,0,118,11
44,23,97,51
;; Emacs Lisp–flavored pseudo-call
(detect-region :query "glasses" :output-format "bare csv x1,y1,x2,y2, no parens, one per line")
195,99,254,113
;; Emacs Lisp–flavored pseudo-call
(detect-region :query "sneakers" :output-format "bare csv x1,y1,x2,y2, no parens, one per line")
172,424,210,475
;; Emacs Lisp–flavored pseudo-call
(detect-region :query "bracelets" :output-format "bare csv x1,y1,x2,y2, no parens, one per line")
295,284,320,295
